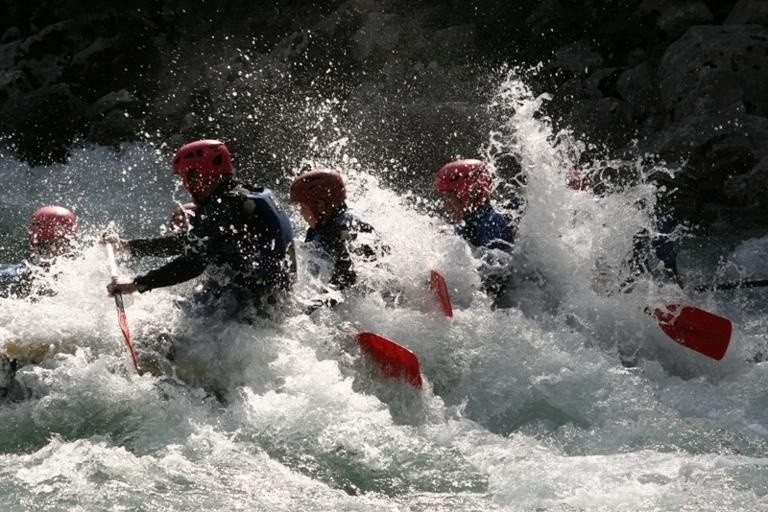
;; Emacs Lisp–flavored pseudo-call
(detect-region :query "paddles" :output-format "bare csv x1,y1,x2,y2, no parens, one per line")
353,332,420,389
429,270,452,316
102,232,137,370
643,305,731,360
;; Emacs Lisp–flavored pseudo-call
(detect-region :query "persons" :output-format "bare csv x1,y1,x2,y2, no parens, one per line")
99,141,297,326
283,170,390,314
0,205,80,299
434,158,515,305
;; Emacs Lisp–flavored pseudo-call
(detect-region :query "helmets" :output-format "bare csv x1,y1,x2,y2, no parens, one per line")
292,169,346,218
434,159,493,212
166,200,196,229
171,139,233,190
26,207,76,244
